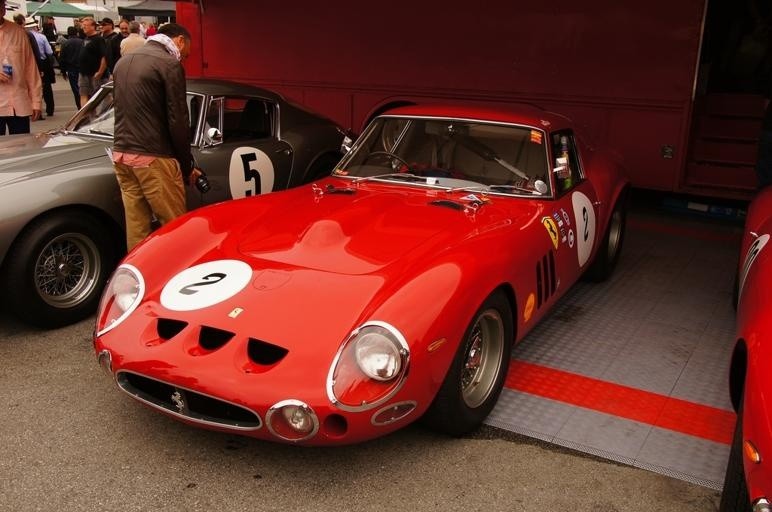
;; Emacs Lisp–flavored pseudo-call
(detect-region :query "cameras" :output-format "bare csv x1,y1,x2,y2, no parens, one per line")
184,167,211,193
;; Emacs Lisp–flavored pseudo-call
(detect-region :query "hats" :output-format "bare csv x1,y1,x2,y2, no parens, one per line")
98,18,113,25
24,17,38,27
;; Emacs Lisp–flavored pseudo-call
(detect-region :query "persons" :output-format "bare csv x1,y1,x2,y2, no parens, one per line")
111,22,201,252
0,0,43,137
14,12,158,122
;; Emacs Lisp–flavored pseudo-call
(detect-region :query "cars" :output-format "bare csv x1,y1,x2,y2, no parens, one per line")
721,182,772,512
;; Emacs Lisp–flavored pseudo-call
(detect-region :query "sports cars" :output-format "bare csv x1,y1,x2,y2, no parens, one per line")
92,95,629,446
1,77,353,331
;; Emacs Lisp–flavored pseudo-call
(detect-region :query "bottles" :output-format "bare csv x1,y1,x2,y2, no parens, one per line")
3,56,13,85
558,136,574,191
190,154,210,194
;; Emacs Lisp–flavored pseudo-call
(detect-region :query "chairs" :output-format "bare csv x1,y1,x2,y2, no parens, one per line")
438,124,544,188
398,120,445,170
225,98,269,144
